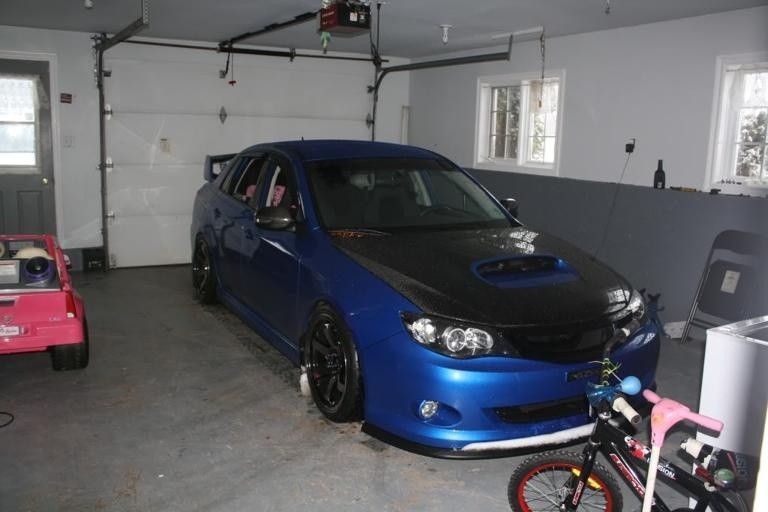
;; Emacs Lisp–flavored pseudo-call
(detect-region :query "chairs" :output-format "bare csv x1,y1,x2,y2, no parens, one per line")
680,229,767,349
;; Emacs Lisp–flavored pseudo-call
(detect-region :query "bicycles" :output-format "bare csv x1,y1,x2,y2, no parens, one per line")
499,309,758,512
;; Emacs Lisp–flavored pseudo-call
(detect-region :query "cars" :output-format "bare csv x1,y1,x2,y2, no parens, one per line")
185,135,666,458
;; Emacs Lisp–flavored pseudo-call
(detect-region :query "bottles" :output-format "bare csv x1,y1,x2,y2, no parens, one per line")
654,160,666,189
